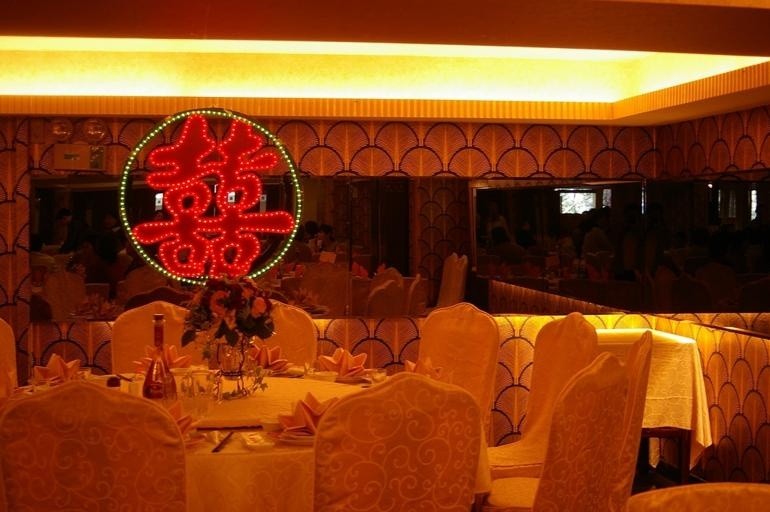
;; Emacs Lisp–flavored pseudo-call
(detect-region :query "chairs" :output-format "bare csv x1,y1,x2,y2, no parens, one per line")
1,301,770,512
31,250,469,319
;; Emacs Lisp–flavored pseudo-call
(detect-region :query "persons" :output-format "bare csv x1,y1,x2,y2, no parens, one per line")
477,202,770,281
34,209,163,285
257,220,340,266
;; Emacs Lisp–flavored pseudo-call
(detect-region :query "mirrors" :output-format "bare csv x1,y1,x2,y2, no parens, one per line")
24,170,769,324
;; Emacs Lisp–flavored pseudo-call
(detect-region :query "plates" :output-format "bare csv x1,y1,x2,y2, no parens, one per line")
168,367,188,374
338,375,363,383
262,430,315,446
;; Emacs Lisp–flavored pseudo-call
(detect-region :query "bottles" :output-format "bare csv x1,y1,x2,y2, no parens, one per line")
142,312,178,415
106,376,139,397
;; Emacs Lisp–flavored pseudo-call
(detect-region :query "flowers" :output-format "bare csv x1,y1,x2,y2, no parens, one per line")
182,275,277,346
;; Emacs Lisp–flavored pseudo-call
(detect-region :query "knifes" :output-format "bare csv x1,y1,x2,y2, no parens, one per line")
211,431,234,454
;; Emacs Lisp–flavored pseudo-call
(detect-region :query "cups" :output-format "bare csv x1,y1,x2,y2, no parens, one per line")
34,379,50,392
371,367,388,386
260,418,281,432
182,371,215,417
315,370,337,382
